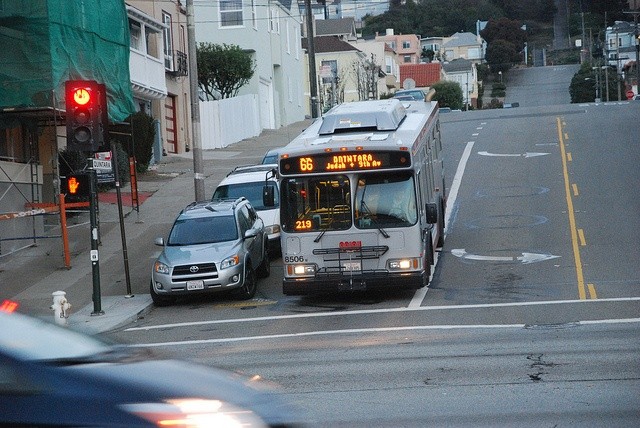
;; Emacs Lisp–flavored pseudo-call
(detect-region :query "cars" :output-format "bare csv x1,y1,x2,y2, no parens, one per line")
393,94,417,101
262,146,285,164
1,309,318,426
395,88,427,99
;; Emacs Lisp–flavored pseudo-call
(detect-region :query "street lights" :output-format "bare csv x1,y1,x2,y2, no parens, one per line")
602,65,610,102
585,77,598,99
608,26,621,102
593,65,599,99
615,19,640,96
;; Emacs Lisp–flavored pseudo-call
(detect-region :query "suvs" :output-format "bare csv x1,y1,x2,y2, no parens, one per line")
150,196,270,307
211,164,282,255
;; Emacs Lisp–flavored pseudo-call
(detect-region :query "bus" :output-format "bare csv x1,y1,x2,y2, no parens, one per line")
279,97,447,295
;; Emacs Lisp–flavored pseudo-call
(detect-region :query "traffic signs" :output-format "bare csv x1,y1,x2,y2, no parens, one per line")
93,160,112,171
96,174,115,184
96,153,113,160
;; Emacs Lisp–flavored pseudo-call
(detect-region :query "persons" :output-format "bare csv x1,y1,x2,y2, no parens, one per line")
382,188,412,223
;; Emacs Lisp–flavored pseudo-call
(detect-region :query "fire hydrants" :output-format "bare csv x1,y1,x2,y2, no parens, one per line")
48,291,72,327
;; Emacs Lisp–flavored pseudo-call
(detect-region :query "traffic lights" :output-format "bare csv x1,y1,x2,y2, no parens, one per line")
98,83,110,153
65,80,99,153
